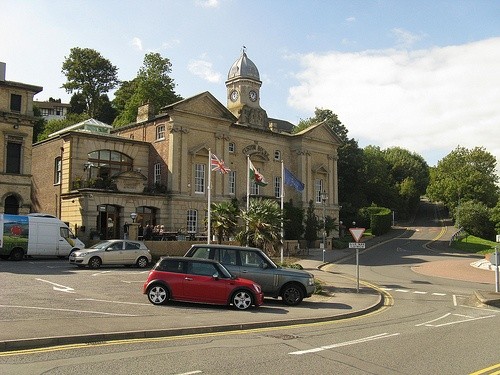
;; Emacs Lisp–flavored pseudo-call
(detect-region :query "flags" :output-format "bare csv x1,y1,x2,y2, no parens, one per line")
284,166,304,192
249,161,268,187
211,155,231,173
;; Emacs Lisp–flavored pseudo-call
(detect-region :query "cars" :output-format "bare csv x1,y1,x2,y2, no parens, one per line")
69,239,151,270
143,256,264,311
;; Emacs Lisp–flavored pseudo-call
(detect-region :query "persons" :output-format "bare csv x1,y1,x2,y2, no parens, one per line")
138,224,165,240
124,222,129,240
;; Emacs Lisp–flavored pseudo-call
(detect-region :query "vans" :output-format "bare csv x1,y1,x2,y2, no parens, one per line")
0,214,85,261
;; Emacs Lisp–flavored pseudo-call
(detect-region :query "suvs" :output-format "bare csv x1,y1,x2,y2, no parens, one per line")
178,244,316,306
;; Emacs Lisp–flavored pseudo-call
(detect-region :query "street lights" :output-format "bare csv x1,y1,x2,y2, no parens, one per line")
320,189,329,254
